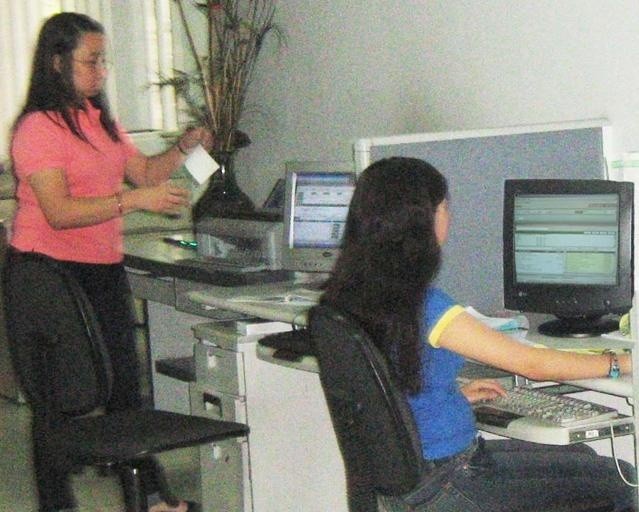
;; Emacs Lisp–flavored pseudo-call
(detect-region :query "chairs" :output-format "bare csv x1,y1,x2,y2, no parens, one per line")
307,305,616,512
3,267,325,512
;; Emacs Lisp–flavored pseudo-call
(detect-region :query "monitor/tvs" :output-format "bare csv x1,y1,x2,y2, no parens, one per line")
281,159,357,301
503,178,636,338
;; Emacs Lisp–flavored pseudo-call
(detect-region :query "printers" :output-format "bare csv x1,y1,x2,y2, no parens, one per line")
175,178,285,276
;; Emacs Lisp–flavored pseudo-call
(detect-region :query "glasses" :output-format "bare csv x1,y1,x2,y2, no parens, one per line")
71,56,112,72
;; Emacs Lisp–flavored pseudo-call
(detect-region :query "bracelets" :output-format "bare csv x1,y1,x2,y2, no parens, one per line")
115,191,124,215
606,350,620,378
176,142,192,157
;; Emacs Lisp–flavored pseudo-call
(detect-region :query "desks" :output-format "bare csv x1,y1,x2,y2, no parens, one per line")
186,266,637,512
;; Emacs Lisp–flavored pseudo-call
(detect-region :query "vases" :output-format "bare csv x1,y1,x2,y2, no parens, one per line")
191,150,255,242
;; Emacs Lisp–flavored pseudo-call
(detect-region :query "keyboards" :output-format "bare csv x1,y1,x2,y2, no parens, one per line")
480,385,618,426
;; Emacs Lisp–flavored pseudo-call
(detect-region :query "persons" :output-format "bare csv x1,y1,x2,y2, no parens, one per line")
313,157,638,512
2,11,204,511
